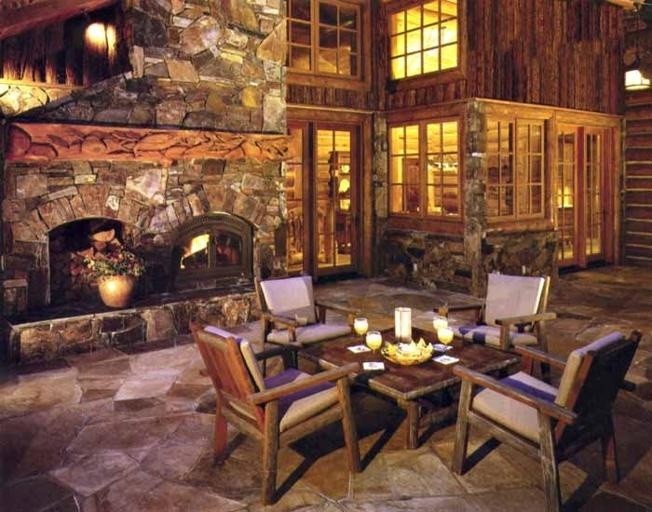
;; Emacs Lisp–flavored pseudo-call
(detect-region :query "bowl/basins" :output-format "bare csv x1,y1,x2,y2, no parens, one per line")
376,337,433,368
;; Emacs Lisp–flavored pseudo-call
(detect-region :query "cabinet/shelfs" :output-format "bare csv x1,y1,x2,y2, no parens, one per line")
330,150,351,254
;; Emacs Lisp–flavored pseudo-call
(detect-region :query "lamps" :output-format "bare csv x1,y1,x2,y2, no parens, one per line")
394,307,411,345
623,68,650,91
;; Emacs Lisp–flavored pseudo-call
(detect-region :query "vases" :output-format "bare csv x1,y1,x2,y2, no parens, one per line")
96,274,137,309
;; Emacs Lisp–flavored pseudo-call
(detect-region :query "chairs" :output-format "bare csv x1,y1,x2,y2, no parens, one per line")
258,275,361,379
451,330,641,511
187,322,362,506
431,271,557,386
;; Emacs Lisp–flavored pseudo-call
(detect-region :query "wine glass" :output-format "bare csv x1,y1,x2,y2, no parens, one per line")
430,317,456,354
353,316,383,357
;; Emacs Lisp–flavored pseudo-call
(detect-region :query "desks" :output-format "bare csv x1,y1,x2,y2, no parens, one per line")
297,325,520,451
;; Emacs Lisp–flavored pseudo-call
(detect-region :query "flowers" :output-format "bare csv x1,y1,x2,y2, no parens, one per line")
82,245,149,281
